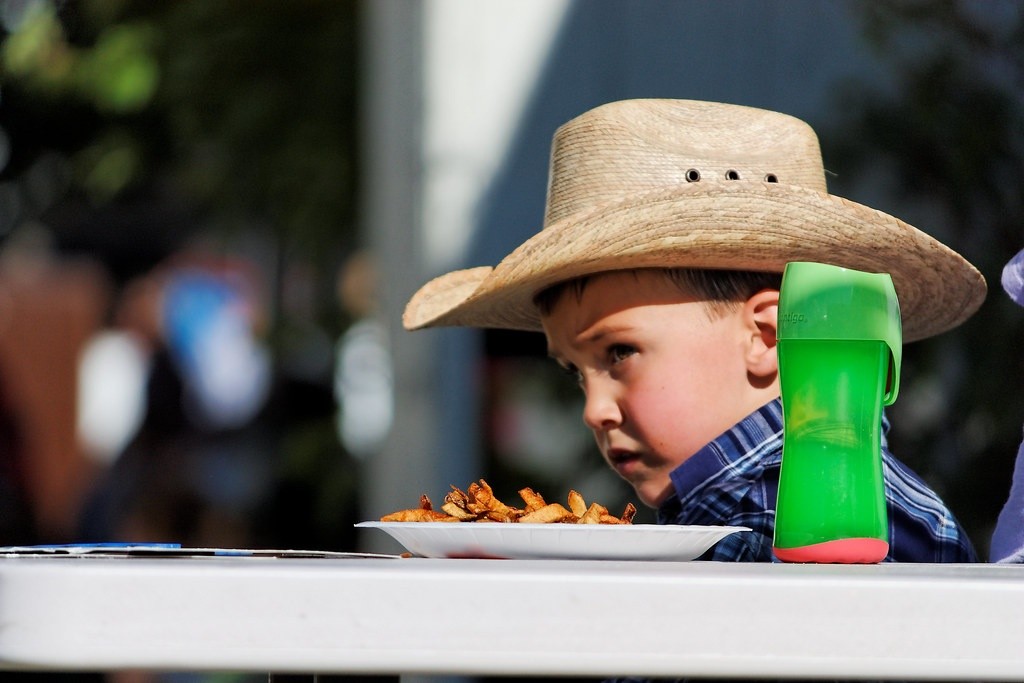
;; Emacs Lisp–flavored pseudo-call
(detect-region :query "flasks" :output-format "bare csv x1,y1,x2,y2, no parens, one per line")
770,260,902,565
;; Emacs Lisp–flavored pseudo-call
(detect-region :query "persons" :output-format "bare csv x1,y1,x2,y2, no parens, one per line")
399,93,987,565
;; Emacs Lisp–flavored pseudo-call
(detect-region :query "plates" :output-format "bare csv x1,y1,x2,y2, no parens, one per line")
355,522,751,561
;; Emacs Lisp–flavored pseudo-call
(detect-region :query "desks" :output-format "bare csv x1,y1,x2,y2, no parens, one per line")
0,548,1024,683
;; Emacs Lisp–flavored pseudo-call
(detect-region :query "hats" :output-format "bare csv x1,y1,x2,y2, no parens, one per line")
403,97,991,348
